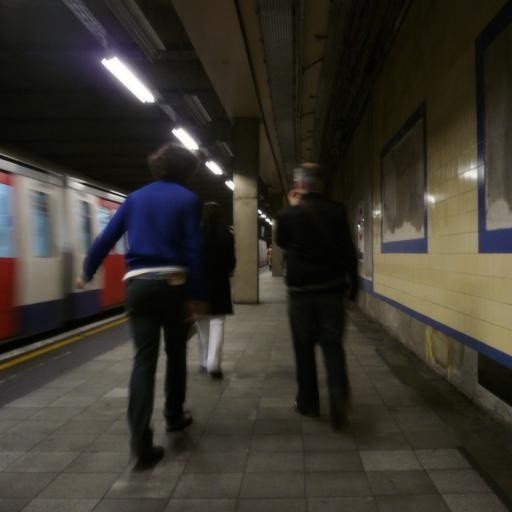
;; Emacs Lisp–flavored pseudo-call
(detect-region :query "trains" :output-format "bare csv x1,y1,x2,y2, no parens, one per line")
0,152,268,355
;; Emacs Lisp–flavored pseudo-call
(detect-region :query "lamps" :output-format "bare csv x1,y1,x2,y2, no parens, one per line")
100,43,157,104
169,120,275,226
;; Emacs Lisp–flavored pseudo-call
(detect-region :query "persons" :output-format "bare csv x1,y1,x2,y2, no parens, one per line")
192,201,236,380
274,156,362,429
264,245,272,271
74,144,202,471
